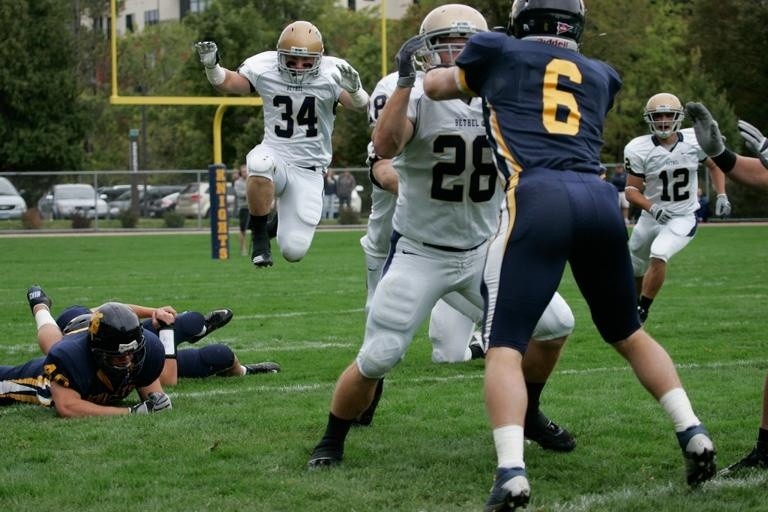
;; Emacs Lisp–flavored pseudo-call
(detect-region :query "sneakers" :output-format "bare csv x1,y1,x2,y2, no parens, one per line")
483,467,532,512
673,423,718,487
251,238,274,268
189,307,233,345
26,283,53,317
716,446,767,479
305,437,345,471
522,404,576,454
469,331,484,355
244,361,281,376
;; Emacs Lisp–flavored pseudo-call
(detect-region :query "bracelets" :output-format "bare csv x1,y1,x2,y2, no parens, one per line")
710,146,737,174
205,64,226,87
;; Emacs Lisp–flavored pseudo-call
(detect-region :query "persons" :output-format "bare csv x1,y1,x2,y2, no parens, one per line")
703,192,714,222
324,167,336,221
612,163,641,224
695,188,706,221
308,3,574,468
58,302,282,384
624,93,731,326
349,142,398,427
0,285,172,418
336,172,355,209
195,22,372,268
231,164,249,256
686,102,768,480
423,0,718,510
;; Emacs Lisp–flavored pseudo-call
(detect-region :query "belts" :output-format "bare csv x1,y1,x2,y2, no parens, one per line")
422,239,487,252
300,165,317,171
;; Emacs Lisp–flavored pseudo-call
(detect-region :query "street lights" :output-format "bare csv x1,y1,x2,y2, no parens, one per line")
126,127,141,218
140,52,155,217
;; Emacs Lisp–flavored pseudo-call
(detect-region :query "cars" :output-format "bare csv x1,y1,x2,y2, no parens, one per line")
36,182,109,221
100,177,243,222
323,171,365,219
0,176,28,222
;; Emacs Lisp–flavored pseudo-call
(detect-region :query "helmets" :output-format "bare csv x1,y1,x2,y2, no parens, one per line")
417,3,490,74
63,313,92,335
276,19,325,88
88,301,147,387
643,92,685,141
505,0,587,41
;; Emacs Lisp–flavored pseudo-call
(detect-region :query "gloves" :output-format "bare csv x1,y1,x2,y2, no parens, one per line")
147,391,173,415
715,193,731,220
194,40,221,69
395,33,425,88
648,203,675,225
128,399,154,416
736,118,767,168
684,100,725,159
330,63,361,95
